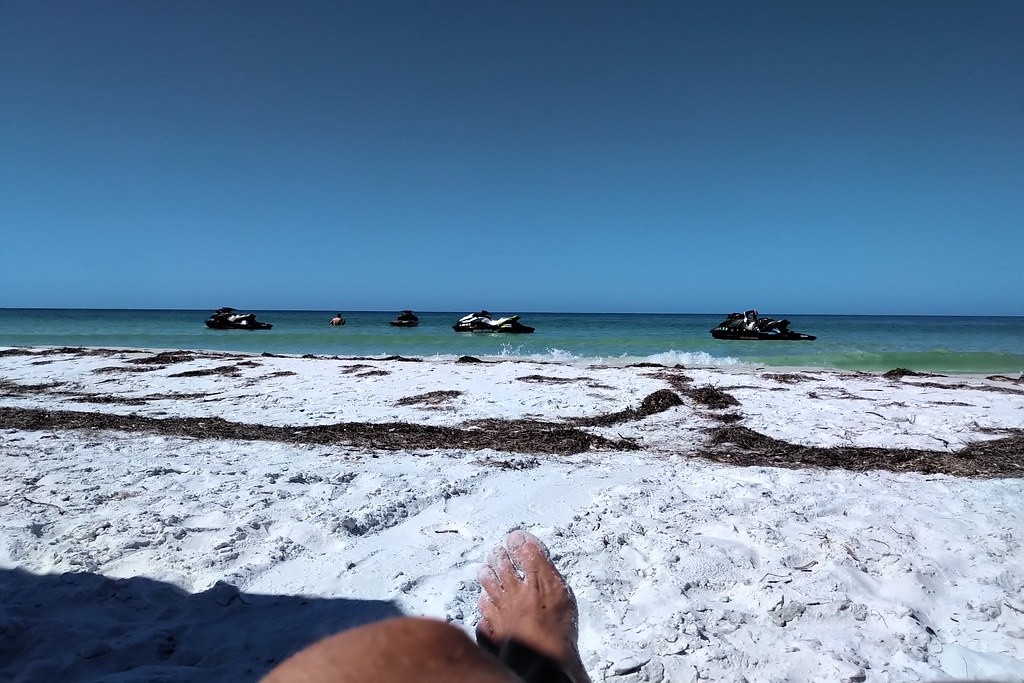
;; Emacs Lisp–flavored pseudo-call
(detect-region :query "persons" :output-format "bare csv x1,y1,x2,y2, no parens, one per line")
329,313,346,326
259,530,594,683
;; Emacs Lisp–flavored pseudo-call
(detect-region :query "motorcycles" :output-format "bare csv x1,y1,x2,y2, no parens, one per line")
204,307,274,330
709,310,817,341
388,310,421,327
452,310,535,333
